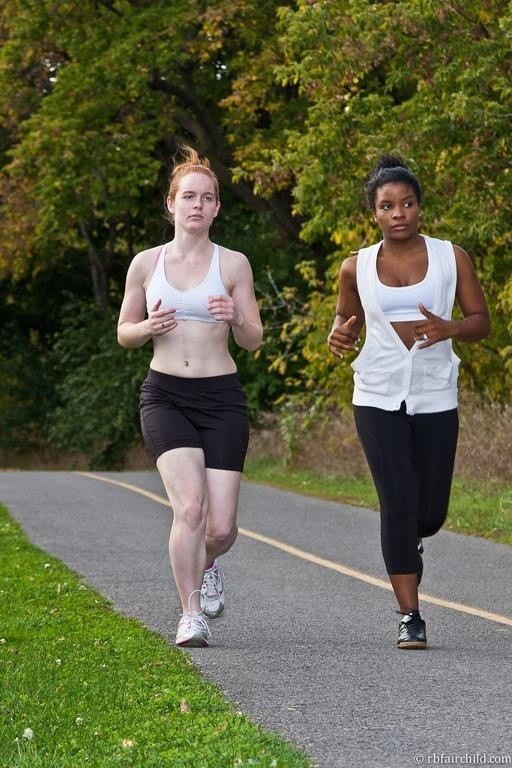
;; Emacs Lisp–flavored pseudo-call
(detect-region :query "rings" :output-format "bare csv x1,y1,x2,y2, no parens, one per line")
162,323,164,328
422,333,428,341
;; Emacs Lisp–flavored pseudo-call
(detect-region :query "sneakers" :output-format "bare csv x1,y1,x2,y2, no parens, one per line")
415,538,424,585
200,560,225,618
397,610,427,649
175,611,210,647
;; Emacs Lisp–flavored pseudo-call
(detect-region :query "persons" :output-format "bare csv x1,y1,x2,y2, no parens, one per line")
116,143,265,646
327,150,492,650
